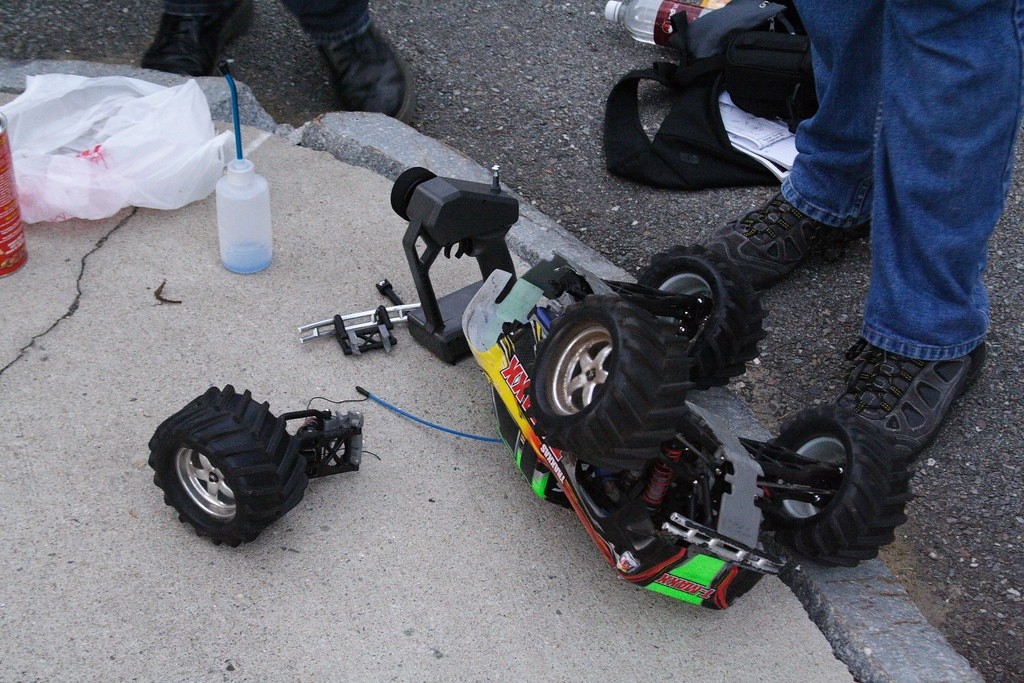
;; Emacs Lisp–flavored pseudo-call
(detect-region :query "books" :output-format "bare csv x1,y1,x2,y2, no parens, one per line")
728,133,799,183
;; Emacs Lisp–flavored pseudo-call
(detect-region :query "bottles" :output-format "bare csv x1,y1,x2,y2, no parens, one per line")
215,159,273,272
604,0,715,49
0,112,28,280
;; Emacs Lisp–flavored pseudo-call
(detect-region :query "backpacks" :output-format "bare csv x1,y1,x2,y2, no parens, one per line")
602,0,819,192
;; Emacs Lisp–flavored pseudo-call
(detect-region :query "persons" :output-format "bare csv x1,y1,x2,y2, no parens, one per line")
696,0,1024,457
142,0,413,124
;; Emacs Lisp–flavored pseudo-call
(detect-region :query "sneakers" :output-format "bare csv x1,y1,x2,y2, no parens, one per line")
819,336,986,462
700,193,871,292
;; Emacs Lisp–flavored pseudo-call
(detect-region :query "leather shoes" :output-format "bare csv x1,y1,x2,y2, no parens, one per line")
316,20,416,125
139,0,254,78
